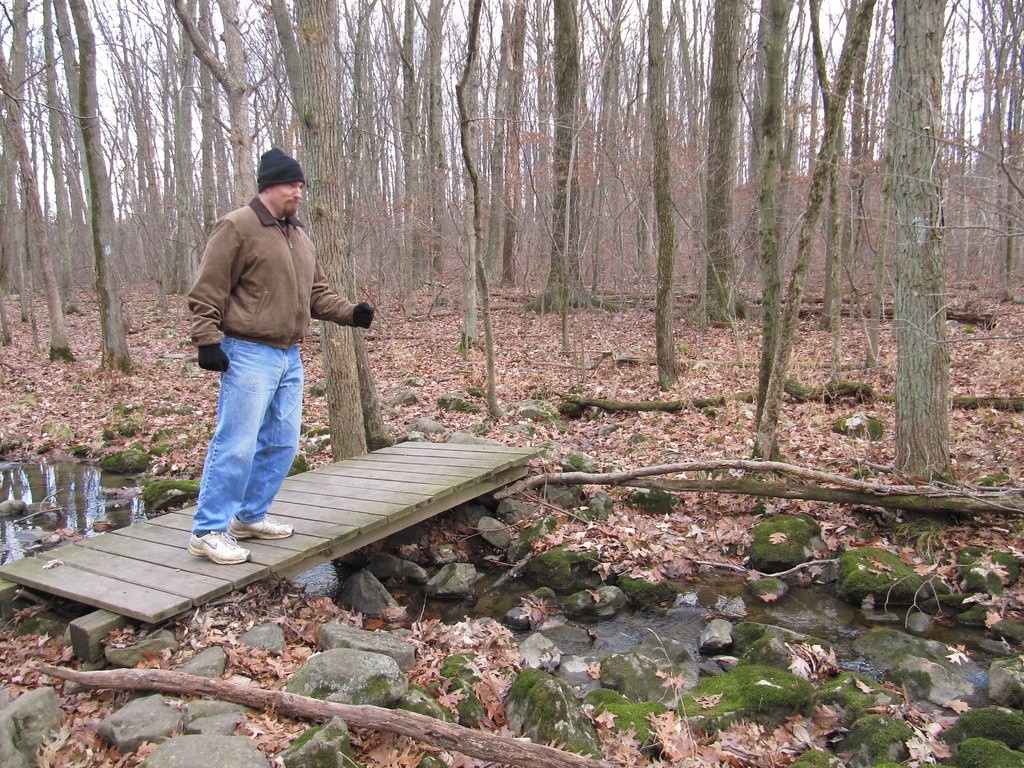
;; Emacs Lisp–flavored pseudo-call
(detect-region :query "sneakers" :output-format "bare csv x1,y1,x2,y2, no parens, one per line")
187,531,251,565
226,515,294,541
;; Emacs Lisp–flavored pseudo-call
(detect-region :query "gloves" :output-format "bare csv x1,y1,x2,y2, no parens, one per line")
353,302,374,329
197,344,229,373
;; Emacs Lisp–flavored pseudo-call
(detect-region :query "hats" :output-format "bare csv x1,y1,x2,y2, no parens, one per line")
257,148,305,193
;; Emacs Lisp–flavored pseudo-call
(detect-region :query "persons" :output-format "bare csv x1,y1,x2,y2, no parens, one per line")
185,146,374,564
